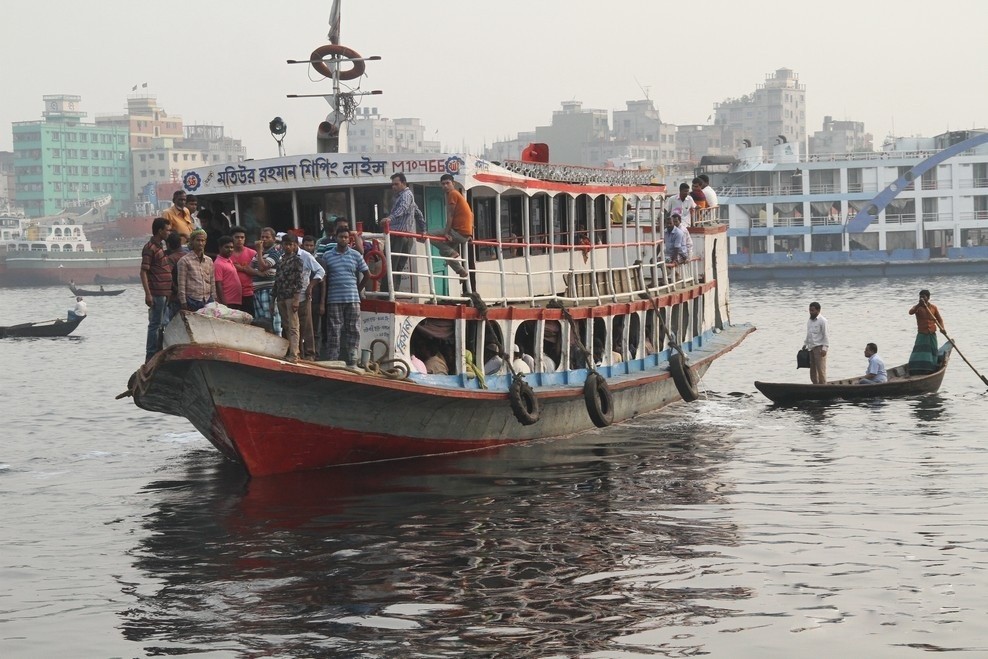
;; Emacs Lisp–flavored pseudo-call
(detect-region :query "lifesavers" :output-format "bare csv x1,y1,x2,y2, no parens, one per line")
583,372,614,428
669,353,699,402
508,381,541,426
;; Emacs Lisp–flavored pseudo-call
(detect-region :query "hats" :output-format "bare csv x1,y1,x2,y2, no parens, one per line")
486,343,499,353
514,344,520,352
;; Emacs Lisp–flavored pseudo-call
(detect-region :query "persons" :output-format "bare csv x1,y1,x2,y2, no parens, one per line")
489,223,602,260
70,279,75,288
737,245,749,254
907,289,946,377
966,235,985,247
645,174,717,288
100,286,104,291
858,343,888,384
428,174,474,284
68,296,87,320
411,327,677,375
610,194,634,224
803,302,829,384
376,172,428,300
140,189,371,370
824,240,832,251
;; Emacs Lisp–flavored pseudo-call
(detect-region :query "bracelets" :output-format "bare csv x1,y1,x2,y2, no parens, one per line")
239,266,245,272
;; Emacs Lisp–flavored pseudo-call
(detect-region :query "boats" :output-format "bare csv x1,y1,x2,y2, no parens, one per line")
125,150,756,479
70,287,127,296
755,339,954,402
0,314,87,336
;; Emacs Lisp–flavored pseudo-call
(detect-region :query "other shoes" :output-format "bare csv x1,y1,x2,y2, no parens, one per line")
286,352,315,361
346,364,366,373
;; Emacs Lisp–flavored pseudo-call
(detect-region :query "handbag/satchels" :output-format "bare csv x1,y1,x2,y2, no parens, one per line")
796,347,810,369
